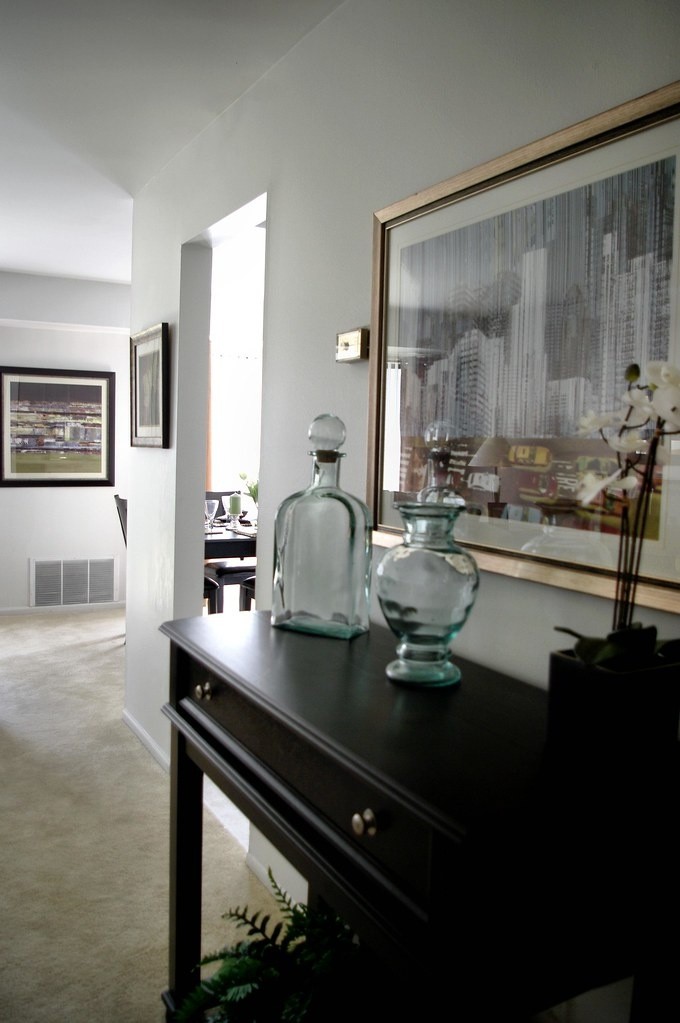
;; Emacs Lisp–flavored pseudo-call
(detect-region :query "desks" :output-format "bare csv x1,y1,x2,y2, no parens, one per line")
205,516,257,558
158,609,680,1023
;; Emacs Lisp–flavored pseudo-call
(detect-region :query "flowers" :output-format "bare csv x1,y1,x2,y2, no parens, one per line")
574,358,680,662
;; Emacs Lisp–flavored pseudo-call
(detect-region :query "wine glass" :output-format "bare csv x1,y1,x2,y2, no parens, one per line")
205,500,219,530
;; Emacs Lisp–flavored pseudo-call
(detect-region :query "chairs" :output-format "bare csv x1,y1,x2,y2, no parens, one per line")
204,492,256,612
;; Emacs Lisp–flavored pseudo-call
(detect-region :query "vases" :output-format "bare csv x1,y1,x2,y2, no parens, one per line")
546,651,680,785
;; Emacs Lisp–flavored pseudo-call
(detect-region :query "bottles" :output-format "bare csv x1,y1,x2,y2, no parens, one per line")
270,414,373,637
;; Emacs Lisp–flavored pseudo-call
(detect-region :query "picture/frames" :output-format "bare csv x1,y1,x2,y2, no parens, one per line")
364,66,680,615
129,320,168,448
0,365,115,489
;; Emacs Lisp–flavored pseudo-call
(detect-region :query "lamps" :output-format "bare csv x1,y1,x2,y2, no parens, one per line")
469,436,516,519
